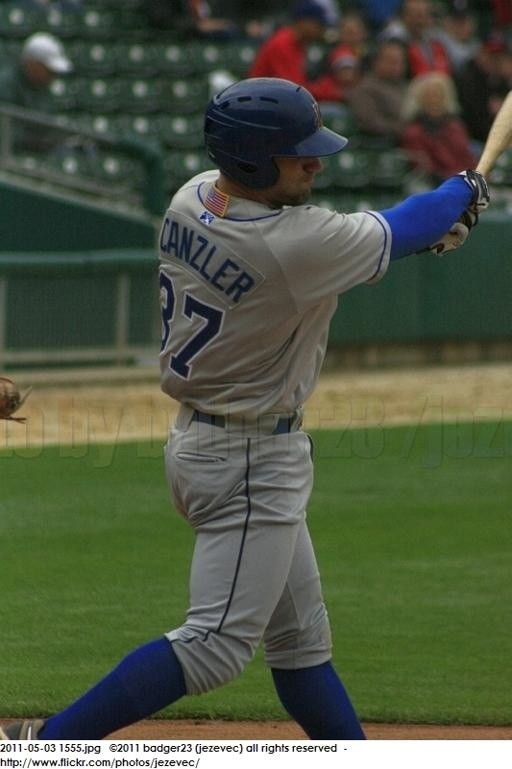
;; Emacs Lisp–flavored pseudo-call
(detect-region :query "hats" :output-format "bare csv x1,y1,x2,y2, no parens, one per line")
22,31,74,75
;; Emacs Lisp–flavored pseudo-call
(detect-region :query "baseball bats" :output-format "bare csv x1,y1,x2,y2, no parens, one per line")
442,87,512,252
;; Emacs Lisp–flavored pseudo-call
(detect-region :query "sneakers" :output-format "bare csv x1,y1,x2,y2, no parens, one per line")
0,719,46,740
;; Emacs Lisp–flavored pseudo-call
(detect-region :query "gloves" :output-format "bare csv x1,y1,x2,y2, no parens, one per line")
415,168,491,257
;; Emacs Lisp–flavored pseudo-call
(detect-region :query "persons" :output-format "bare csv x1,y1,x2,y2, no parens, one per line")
134,0,511,209
0,76,488,740
1,33,74,174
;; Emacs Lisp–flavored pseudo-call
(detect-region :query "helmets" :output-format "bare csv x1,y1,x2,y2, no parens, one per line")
203,77,349,190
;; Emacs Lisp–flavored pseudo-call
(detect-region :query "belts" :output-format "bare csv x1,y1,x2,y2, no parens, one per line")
189,402,298,437
